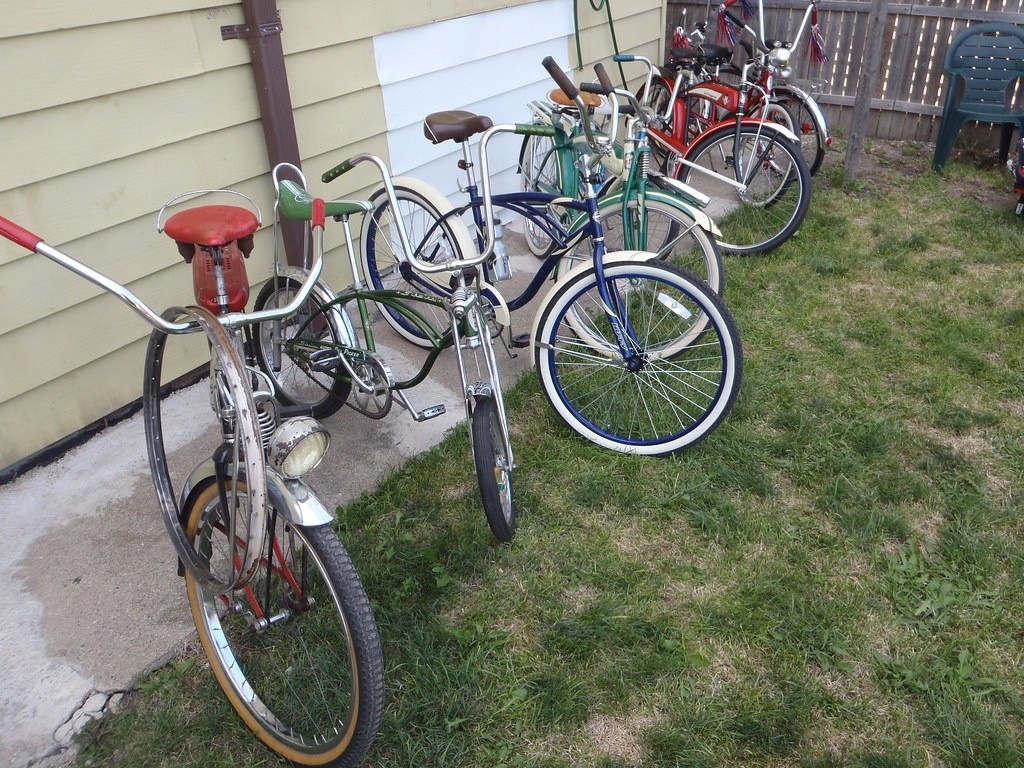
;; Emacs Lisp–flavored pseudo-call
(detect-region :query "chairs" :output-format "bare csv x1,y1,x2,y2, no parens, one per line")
932,21,1024,175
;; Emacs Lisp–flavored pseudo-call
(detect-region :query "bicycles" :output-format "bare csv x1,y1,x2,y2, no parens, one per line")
0,187,386,768
249,0,831,462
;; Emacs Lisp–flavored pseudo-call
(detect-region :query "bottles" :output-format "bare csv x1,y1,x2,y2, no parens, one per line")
486,219,512,282
